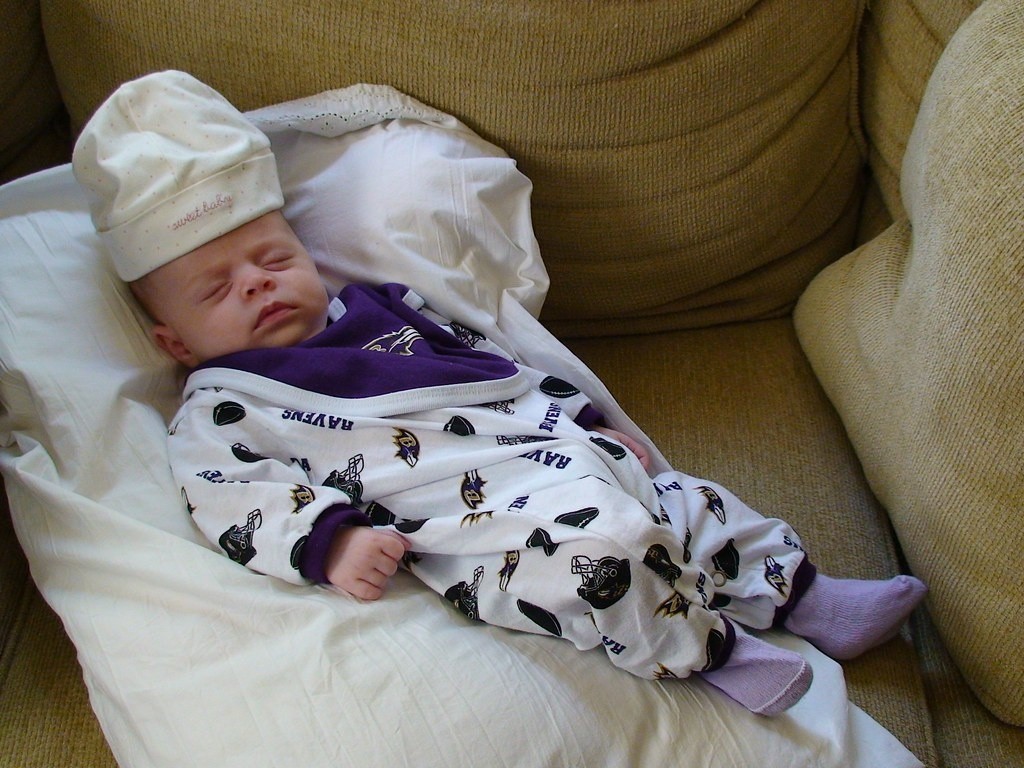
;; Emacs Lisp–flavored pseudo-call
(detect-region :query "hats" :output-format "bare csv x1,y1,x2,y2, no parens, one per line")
71,70,284,282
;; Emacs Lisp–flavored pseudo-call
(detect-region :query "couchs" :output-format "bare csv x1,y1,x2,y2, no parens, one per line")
0,0,1024,768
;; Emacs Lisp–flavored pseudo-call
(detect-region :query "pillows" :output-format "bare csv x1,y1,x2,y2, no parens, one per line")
791,0,1024,729
38,0,873,339
0,84,930,767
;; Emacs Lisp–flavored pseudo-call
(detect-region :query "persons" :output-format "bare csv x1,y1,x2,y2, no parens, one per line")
71,69,927,717
